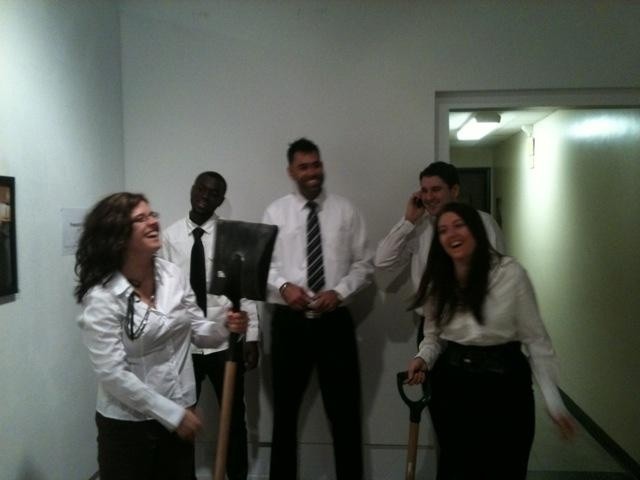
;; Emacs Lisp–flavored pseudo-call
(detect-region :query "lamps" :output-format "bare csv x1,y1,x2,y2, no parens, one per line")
456,111,502,142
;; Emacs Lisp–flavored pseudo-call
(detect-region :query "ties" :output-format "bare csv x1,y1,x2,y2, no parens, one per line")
305,199,325,295
190,226,206,316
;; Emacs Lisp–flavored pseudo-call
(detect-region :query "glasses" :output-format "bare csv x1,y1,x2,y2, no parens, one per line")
131,211,158,223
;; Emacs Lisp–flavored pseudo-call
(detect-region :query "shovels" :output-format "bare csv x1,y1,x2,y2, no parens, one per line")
210,220,279,479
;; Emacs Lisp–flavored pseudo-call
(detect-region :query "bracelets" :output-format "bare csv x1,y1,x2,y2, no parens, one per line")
278,281,289,294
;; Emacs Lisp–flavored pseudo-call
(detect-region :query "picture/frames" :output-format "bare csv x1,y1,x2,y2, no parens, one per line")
0,175,19,299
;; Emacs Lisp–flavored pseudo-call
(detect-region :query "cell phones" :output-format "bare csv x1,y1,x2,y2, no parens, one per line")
413,198,421,209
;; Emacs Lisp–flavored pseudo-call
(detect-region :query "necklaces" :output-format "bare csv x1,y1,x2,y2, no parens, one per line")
122,278,157,341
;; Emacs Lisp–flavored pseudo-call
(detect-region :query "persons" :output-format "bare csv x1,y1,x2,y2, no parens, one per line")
155,170,264,480
369,159,509,444
404,199,580,480
75,192,250,480
260,138,376,480
